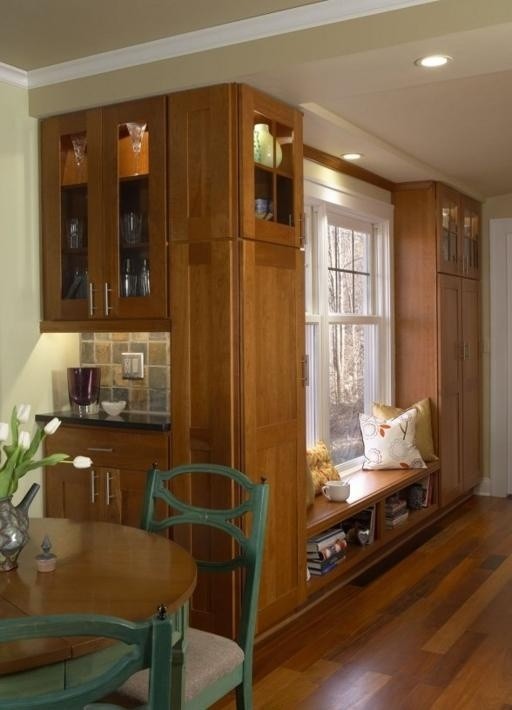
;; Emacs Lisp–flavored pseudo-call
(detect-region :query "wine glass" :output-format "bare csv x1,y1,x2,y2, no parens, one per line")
68,119,146,184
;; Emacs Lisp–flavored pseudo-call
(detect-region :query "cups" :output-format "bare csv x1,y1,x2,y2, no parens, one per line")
63,212,151,299
321,481,351,503
67,364,102,417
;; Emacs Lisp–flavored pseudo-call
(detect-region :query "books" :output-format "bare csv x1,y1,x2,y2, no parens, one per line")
305,501,380,575
385,472,437,530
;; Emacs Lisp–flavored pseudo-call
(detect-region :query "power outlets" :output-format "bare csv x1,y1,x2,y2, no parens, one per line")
122,354,131,381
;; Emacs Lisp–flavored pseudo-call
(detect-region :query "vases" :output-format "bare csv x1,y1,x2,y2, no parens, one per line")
252,124,282,168
0,505,30,572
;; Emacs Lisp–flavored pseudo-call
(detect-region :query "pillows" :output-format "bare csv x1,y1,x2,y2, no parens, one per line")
307,436,342,509
357,408,427,473
371,397,439,462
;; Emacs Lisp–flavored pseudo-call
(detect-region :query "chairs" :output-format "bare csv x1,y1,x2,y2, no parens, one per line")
1,597,179,710
115,452,275,710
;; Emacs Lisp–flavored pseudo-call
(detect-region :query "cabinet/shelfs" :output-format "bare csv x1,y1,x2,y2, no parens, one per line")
397,181,483,508
306,468,445,595
43,419,174,543
36,96,169,335
170,239,309,639
168,83,305,248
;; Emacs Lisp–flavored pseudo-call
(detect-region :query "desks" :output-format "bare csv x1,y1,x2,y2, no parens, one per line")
0,510,205,680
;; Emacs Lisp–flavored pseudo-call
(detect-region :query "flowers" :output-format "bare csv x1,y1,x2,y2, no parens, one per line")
0,395,96,501
